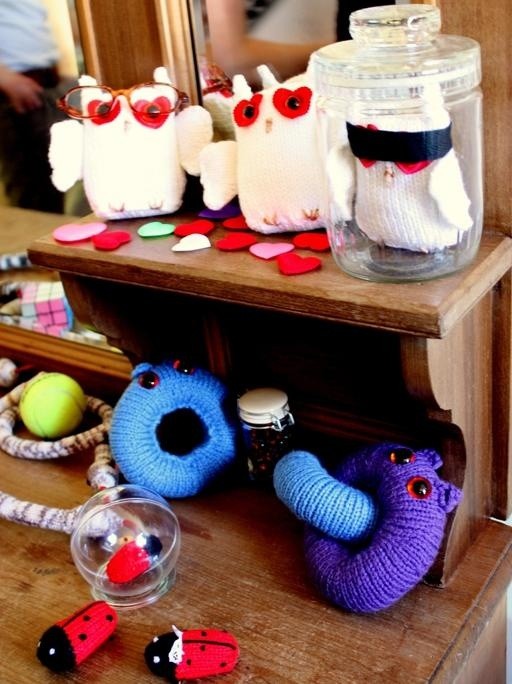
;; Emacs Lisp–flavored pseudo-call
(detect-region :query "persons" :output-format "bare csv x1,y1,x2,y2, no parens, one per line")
194,0,394,99
0,0,66,213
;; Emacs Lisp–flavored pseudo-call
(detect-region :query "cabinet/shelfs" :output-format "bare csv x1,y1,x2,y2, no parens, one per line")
234,386,298,486
310,1,485,286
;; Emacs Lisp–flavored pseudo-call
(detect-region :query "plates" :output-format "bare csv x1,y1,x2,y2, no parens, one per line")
0,211,511,684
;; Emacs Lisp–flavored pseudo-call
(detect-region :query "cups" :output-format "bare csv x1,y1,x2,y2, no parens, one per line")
55,81,189,120
